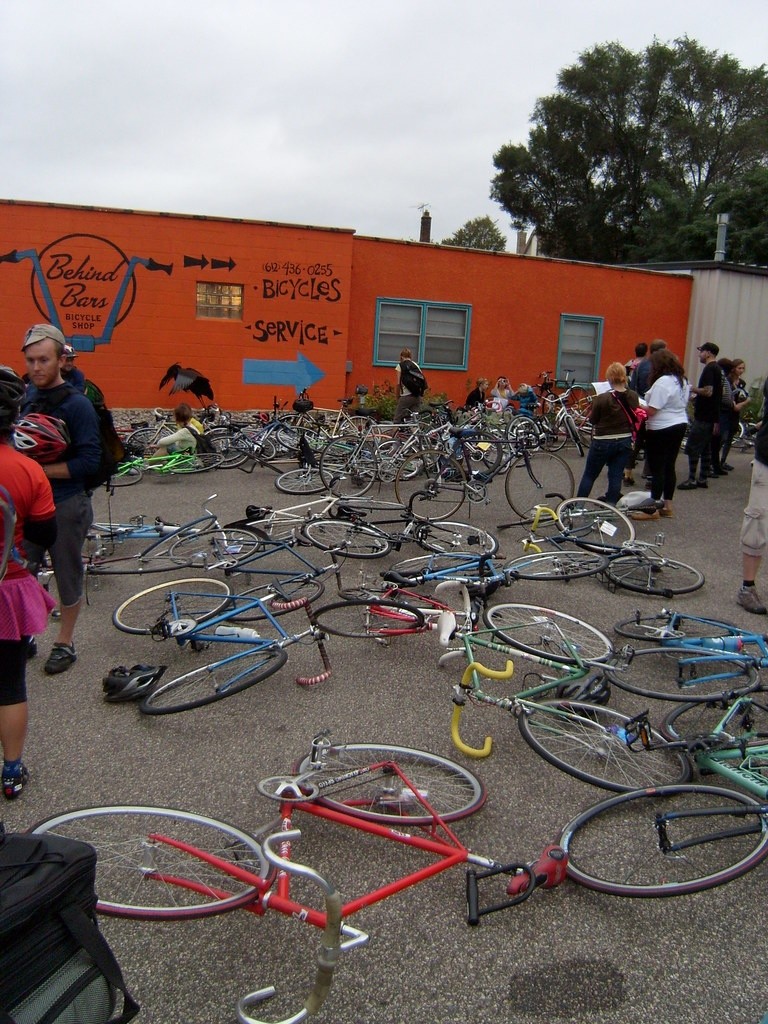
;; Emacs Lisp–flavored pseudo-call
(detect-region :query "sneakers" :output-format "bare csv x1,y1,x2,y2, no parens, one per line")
44,640,76,674
28,638,37,658
735,586,767,615
0,762,29,799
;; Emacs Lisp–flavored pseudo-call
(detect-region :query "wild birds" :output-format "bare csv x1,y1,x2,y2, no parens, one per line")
158,360,214,409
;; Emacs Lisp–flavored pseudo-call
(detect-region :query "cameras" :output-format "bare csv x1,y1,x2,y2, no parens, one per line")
501,379,507,384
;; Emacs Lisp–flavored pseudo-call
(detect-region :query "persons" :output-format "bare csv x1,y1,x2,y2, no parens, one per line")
574,339,749,521
9,324,103,675
129,403,204,475
0,366,56,800
392,347,422,438
465,376,539,426
736,376,768,614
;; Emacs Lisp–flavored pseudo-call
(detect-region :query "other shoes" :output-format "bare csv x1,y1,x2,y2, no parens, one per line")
632,509,661,522
709,467,728,479
721,463,735,472
644,481,652,492
623,477,635,487
696,479,709,488
677,480,698,489
660,508,673,518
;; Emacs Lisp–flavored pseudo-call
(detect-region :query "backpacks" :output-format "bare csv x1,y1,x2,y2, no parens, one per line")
399,358,429,397
29,386,127,498
183,426,218,466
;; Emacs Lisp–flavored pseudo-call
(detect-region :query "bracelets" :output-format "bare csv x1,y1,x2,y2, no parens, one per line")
742,402,745,405
394,394,399,397
691,387,695,392
43,465,47,473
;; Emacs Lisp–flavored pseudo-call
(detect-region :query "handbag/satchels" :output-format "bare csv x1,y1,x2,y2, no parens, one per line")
0,830,139,1023
632,423,640,443
630,392,650,422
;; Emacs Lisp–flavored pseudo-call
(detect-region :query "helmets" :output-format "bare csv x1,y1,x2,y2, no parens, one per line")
103,663,167,703
12,413,71,465
0,365,27,417
555,672,612,719
77,380,104,406
459,569,500,599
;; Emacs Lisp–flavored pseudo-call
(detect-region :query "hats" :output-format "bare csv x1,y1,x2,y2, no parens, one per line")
697,342,720,357
21,323,66,352
66,344,80,357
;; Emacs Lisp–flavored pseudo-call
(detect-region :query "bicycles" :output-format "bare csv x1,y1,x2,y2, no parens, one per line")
167,476,407,567
166,527,342,622
436,603,694,794
602,609,768,702
730,411,757,452
24,728,568,1024
111,573,332,715
35,552,191,606
379,531,608,602
300,489,499,562
681,419,744,450
553,682,768,899
516,493,705,598
85,494,228,560
102,369,595,523
496,490,664,531
313,571,480,648
